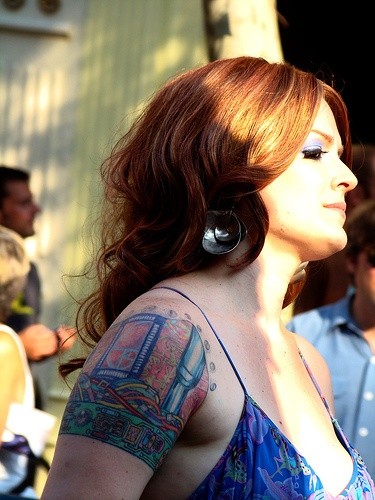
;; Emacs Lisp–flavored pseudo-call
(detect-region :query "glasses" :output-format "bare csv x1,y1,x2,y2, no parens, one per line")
357,253,375,268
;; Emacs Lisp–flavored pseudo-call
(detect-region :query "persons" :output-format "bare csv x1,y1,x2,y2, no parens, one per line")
0,55,375,499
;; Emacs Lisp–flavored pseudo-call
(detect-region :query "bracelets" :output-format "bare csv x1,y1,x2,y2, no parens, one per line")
53,330,62,353
